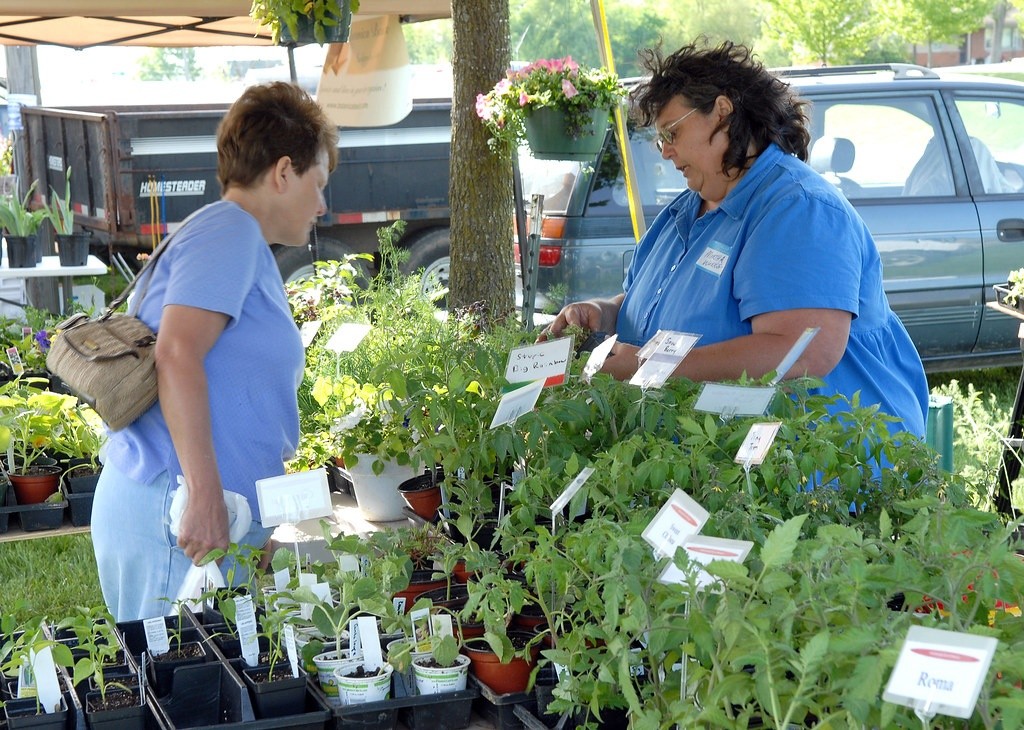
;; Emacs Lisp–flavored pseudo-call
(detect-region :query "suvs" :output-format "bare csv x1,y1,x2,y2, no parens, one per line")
527,62,1023,377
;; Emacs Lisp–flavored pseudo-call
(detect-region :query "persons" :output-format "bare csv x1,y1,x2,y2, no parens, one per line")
531,40,930,513
90,82,336,622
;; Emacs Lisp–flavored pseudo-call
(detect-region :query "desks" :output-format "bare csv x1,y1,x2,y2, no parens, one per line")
0,255,108,316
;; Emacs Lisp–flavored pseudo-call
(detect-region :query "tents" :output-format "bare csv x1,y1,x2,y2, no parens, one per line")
0,0,538,336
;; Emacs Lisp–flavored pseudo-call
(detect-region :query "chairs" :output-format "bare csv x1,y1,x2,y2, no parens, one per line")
809,136,861,190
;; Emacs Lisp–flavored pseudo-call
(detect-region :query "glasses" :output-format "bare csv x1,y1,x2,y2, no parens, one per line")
655,107,697,153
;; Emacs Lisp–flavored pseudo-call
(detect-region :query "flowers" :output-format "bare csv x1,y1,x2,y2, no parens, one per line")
475,54,629,158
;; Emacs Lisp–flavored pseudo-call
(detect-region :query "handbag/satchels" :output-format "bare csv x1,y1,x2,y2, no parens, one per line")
45,205,214,433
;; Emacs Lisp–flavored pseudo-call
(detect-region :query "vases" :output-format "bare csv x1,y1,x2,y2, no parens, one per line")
524,103,610,161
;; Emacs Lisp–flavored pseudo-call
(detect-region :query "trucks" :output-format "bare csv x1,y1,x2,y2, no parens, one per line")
17,95,452,309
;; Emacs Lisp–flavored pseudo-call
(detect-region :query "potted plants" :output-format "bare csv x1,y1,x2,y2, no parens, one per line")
249,0,360,48
0,167,1024,730
